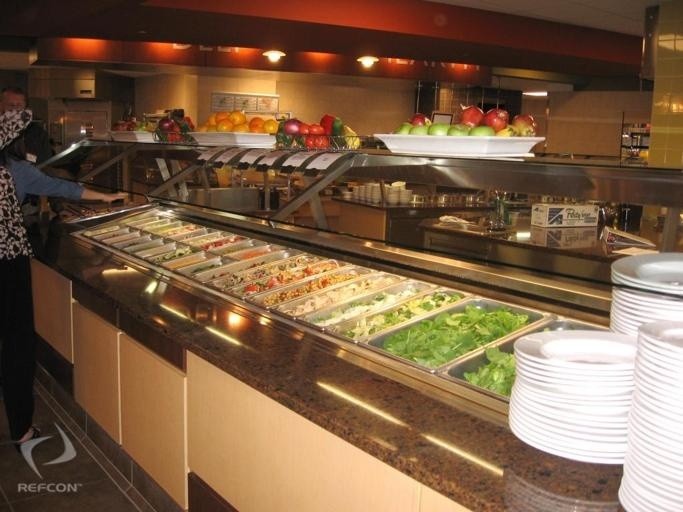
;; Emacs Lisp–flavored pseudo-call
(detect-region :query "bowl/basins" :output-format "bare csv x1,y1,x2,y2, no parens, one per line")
352,180,415,206
342,191,353,199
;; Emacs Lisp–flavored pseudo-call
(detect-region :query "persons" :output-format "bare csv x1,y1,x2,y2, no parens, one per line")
0,108,41,453
0,137,130,208
0,85,74,164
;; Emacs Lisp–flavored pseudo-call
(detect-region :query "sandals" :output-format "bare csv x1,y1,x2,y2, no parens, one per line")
14,424,41,454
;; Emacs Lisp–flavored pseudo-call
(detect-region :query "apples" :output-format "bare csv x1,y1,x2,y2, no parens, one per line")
410,113,432,126
333,116,342,136
484,108,509,131
391,122,496,137
459,103,484,126
512,113,539,136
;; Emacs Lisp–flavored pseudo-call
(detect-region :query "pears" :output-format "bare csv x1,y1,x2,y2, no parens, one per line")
343,125,360,149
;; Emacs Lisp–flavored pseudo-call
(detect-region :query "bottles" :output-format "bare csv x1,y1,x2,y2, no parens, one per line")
500,192,510,225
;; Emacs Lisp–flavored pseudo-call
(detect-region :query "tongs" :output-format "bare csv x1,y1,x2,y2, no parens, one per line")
64,204,85,219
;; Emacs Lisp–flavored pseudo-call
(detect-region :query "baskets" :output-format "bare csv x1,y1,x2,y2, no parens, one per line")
152,130,197,145
276,134,370,153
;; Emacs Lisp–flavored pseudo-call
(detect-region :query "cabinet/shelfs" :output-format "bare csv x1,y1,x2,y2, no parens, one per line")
70,299,189,510
27,68,95,99
619,113,650,160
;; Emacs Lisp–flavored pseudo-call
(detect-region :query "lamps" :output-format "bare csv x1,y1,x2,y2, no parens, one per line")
356,54,380,68
263,47,286,63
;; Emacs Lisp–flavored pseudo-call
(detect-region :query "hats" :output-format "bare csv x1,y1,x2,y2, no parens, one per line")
0,109,33,151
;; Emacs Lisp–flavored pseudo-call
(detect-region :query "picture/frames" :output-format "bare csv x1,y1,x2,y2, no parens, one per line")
431,111,455,125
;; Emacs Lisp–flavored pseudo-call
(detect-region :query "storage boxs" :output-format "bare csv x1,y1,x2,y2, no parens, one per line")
530,203,600,229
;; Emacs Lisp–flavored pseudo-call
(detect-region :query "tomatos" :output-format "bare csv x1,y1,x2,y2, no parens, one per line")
299,124,328,148
321,115,334,133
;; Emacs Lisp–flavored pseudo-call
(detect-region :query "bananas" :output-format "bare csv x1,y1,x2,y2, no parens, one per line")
496,126,532,136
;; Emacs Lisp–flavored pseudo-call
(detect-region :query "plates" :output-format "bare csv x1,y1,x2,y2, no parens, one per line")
610,253,683,331
617,321,683,512
501,465,621,512
508,330,636,466
